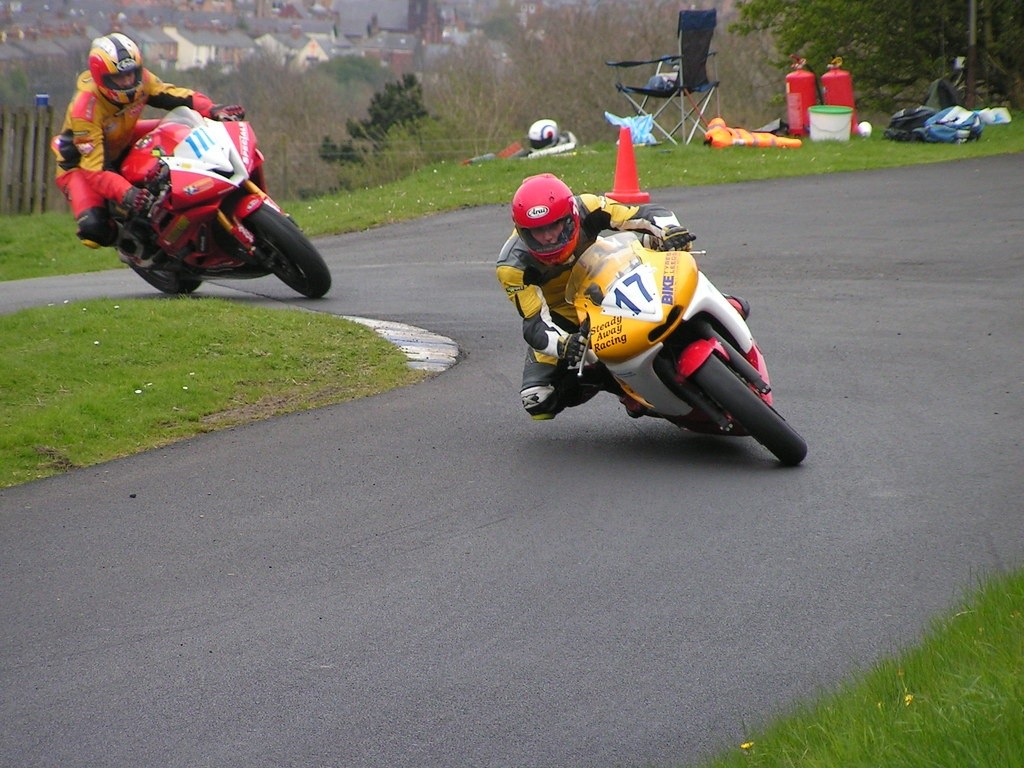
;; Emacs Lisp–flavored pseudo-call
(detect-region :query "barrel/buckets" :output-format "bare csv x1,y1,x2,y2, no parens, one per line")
808,105,854,142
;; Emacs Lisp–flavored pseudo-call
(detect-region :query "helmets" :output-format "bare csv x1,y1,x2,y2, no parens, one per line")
511,173,580,266
89,33,143,104
528,119,558,153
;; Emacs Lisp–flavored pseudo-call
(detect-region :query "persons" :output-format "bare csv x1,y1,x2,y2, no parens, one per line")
496,174,751,423
525,120,568,157
52,34,268,267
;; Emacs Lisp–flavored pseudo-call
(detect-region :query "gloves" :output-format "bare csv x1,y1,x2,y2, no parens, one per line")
557,333,587,362
123,187,157,215
660,224,692,252
210,104,245,121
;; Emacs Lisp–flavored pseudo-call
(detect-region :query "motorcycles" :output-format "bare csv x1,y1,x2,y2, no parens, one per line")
104,111,333,297
563,229,809,469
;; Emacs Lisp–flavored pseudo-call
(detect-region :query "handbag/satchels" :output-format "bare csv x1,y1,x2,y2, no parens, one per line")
604,111,657,144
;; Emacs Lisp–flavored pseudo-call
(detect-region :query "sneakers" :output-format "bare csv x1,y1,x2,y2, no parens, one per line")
726,296,750,321
620,392,648,416
108,221,144,264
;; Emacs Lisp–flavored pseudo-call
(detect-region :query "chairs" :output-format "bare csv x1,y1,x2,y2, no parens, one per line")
605,7,720,147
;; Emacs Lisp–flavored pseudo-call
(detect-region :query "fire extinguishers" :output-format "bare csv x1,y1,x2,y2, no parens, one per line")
820,52,859,134
785,53,820,137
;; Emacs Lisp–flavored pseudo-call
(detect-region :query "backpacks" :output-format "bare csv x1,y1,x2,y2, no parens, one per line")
884,107,935,142
911,105,983,144
